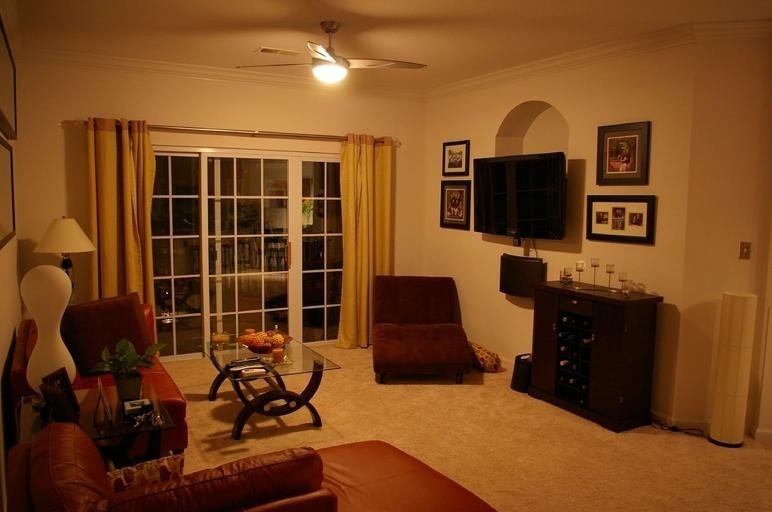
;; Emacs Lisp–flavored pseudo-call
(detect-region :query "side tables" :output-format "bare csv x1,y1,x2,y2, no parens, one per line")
18,381,176,469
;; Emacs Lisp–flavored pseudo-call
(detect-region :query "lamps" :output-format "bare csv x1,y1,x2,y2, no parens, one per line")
707,292,757,449
34,214,98,290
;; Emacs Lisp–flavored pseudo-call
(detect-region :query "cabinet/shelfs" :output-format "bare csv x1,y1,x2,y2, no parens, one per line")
527,279,663,432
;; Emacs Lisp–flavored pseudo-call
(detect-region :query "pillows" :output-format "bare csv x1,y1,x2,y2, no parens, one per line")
106,454,184,493
65,293,154,370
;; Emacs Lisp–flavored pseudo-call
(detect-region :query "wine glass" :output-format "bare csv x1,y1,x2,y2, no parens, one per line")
558,256,629,293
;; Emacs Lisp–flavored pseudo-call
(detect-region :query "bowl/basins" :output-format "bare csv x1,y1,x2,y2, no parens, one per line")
237,330,292,354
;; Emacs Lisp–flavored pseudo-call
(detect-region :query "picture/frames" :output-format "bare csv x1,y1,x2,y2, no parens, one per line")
438,140,472,231
585,193,656,244
595,120,650,186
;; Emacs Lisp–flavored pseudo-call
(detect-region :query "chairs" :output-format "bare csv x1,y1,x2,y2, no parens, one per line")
369,274,471,383
8,303,189,475
263,259,335,324
7,420,497,512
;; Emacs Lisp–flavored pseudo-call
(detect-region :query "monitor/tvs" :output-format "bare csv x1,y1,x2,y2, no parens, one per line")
472,152,567,241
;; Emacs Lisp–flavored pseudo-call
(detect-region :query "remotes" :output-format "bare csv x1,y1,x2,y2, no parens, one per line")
230,356,261,365
240,368,266,376
226,360,264,369
229,363,264,373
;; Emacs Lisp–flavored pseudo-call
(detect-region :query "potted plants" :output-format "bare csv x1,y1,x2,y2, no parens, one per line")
86,336,169,400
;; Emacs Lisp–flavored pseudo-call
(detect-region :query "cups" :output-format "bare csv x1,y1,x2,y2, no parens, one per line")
245,328,255,336
271,348,283,363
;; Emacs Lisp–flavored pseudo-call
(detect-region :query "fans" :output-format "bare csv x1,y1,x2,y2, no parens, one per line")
234,20,427,74
